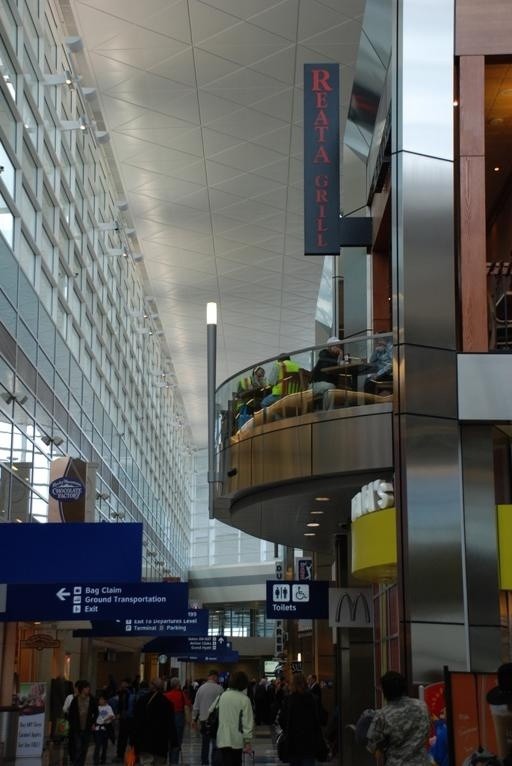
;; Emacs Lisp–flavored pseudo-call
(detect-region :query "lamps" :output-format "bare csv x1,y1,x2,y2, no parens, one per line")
4,385,185,578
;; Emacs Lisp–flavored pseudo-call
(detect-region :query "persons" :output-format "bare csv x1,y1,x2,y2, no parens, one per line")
235,366,271,432
309,337,349,411
363,329,394,405
260,353,308,421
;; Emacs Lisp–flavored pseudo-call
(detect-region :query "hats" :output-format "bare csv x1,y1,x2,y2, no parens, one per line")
327,336,339,343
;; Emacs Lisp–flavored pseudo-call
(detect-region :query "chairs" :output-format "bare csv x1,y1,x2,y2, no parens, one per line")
221,349,396,442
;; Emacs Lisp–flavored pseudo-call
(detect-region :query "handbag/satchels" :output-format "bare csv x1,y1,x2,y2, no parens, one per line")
207,707,219,727
57,719,69,736
184,706,191,722
